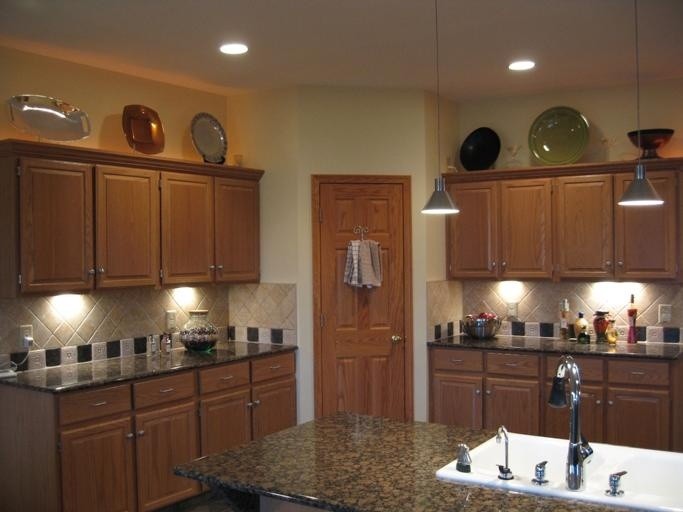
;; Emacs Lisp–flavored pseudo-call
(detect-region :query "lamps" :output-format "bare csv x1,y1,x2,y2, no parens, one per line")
420,1,460,215
617,1,665,208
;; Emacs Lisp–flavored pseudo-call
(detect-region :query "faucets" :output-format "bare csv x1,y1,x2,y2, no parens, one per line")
547,353,594,492
498,425,515,480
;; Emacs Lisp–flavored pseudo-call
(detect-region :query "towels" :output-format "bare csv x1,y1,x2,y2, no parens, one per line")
343,239,363,289
358,239,384,289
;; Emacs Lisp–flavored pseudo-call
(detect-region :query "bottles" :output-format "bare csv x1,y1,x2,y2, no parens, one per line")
558,293,638,345
161,332,172,355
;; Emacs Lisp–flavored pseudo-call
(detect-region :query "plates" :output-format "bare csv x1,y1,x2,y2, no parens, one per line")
460,127,500,170
4,94,91,142
529,106,590,164
122,104,165,154
190,111,227,161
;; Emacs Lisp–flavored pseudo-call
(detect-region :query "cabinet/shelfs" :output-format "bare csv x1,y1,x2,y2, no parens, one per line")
430,346,541,437
158,169,260,286
0,152,157,301
0,367,201,512
542,354,683,451
553,171,679,280
198,351,297,493
450,177,554,278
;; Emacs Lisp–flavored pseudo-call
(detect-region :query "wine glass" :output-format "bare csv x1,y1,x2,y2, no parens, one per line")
627,129,674,159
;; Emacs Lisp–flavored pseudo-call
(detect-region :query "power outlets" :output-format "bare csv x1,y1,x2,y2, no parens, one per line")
658,304,673,323
18,325,34,349
165,310,177,330
506,302,519,320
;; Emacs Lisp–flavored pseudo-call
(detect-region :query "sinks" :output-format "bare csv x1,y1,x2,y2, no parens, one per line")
435,431,683,512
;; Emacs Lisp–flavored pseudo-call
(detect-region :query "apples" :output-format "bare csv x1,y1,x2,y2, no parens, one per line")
477,312,496,319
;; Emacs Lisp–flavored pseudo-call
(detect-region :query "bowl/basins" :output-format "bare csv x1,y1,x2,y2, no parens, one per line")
463,317,503,339
